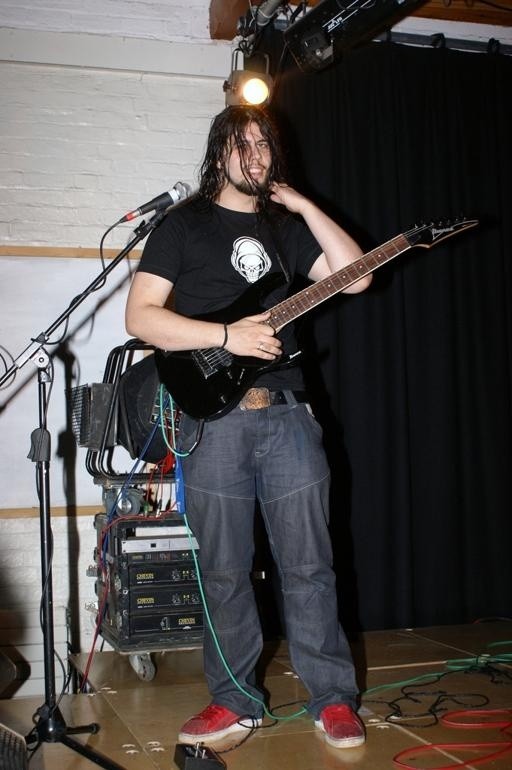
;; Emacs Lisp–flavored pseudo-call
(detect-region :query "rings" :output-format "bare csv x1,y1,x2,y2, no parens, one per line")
259,341,264,350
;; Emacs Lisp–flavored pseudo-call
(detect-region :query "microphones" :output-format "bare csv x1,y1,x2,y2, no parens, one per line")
119,179,194,220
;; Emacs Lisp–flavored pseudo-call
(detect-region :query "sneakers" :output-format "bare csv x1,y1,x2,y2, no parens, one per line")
314,703,366,749
178,702,262,744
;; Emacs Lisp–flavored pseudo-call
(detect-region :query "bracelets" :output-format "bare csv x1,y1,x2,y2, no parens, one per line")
221,321,230,350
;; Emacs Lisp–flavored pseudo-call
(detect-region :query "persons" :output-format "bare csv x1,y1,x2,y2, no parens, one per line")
123,104,377,750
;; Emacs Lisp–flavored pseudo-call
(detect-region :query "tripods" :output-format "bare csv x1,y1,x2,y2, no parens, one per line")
25,375,127,770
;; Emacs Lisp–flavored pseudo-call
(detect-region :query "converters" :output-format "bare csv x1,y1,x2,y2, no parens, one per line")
174,742,228,769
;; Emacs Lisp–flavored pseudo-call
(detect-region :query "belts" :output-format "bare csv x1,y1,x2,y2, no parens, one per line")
234,387,310,412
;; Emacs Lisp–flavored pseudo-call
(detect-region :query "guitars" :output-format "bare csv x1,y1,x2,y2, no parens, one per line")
154,212,480,422
